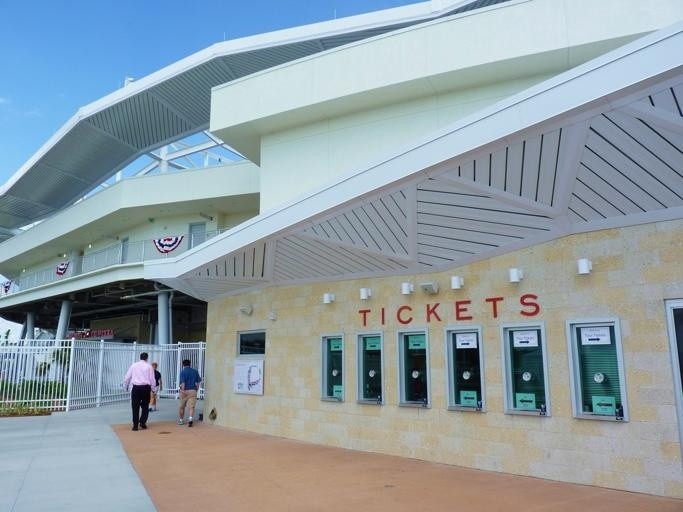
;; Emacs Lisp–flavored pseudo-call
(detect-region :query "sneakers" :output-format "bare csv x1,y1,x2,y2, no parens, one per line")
177,420,193,427
132,422,147,430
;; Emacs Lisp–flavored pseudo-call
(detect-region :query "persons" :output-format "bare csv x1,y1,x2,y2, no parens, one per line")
123,351,156,431
177,359,201,427
148,362,162,411
0,328,11,346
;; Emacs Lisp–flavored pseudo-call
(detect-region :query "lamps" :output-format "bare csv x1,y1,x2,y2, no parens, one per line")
322,258,593,304
